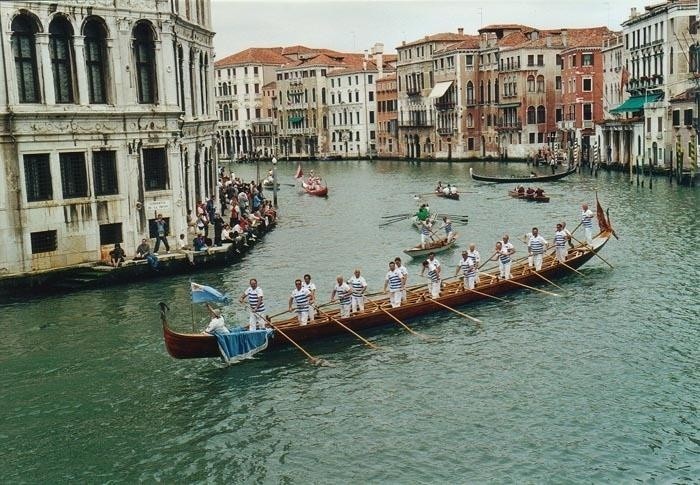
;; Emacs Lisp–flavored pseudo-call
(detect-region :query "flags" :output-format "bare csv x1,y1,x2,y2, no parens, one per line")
597,200,618,240
295,164,304,179
190,282,231,307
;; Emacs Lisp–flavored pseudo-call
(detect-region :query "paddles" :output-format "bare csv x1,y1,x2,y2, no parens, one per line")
378,213,416,228
486,195,513,201
457,191,480,193
278,183,295,187
414,192,442,197
546,193,564,196
434,213,468,226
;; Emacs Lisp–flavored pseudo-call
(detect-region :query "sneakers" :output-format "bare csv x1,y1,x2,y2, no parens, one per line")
235,232,257,253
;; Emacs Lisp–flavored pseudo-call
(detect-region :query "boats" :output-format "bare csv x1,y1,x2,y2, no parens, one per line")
403,233,457,258
434,186,460,199
298,181,330,197
509,188,551,203
468,165,579,183
159,190,612,359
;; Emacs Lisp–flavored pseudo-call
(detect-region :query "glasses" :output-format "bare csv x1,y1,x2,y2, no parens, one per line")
296,282,300,284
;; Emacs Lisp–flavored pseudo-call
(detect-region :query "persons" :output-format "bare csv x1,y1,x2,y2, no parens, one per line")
466,243,480,284
177,151,278,265
304,169,324,191
528,228,549,271
518,233,534,266
345,270,367,313
553,223,568,263
512,184,547,200
110,242,126,268
499,235,515,255
240,279,265,330
456,250,474,290
527,145,568,174
420,252,441,293
288,279,314,327
394,257,408,304
414,181,459,251
153,214,171,256
384,262,404,308
331,275,354,318
491,242,511,278
423,260,440,300
560,221,574,248
582,204,594,244
136,238,150,260
204,303,230,334
300,274,317,318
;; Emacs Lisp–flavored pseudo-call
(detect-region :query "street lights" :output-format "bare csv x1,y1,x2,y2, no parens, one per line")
270,156,278,205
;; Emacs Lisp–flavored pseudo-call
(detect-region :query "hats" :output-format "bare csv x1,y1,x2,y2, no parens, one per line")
212,309,221,316
